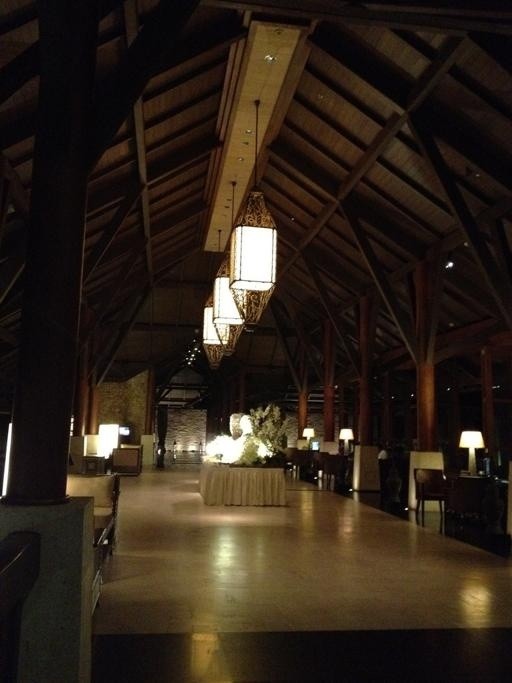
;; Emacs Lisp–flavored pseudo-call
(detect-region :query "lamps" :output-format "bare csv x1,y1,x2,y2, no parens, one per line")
195,91,284,370
458,429,486,475
303,427,315,447
338,428,355,455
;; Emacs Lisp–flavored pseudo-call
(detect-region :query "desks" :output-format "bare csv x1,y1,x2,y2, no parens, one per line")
442,470,510,557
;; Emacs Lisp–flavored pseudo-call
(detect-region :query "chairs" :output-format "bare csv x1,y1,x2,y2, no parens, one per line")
413,468,449,534
110,442,143,476
66,458,121,556
